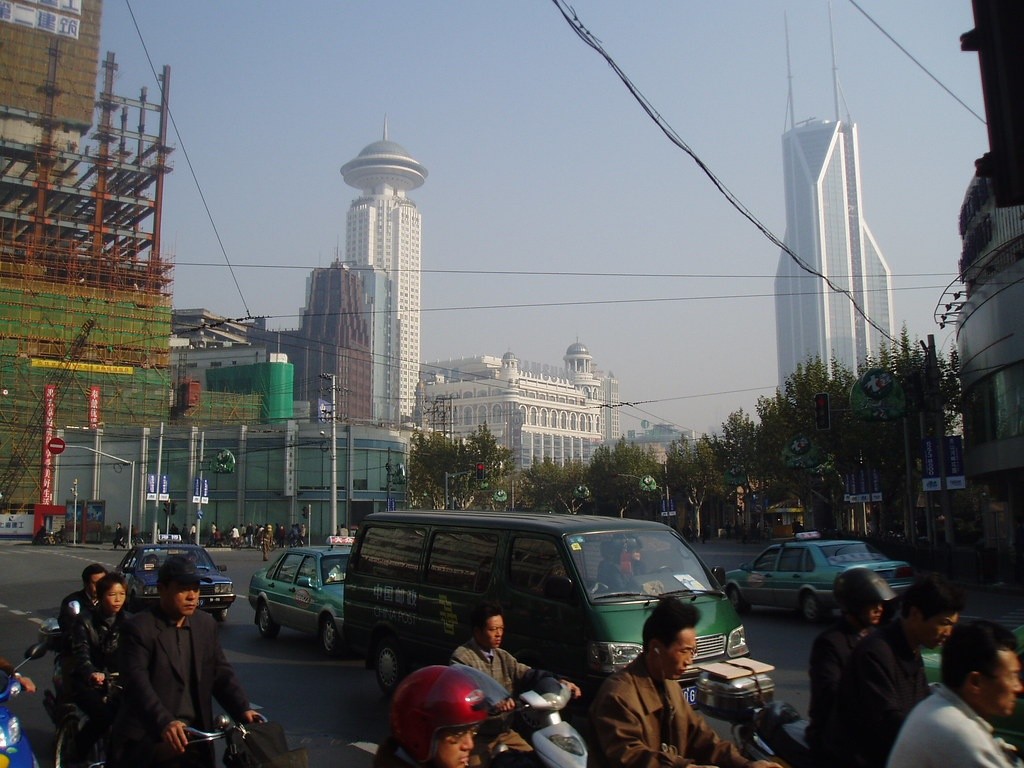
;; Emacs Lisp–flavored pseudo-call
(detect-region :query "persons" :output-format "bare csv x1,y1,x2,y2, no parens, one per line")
702,522,711,545
580,597,782,768
229,522,266,550
114,522,125,549
337,524,356,537
0,655,36,696
725,520,770,544
55,555,268,768
57,525,64,544
35,524,46,544
805,569,1024,768
275,522,307,548
171,523,197,546
792,519,803,535
208,522,222,547
686,521,696,544
389,607,581,768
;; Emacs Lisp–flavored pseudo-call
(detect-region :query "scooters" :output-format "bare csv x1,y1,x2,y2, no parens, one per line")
487,681,589,768
695,656,822,768
1,636,54,768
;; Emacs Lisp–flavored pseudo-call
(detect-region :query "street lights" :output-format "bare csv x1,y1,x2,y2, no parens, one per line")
320,430,339,536
617,473,664,523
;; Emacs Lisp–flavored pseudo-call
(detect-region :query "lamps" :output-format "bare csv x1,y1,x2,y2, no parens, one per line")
984,263,1000,274
945,301,967,311
940,312,963,322
953,290,971,301
940,320,960,330
968,278,984,287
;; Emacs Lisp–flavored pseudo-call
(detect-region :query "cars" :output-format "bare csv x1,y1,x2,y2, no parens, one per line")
111,533,237,622
916,619,1024,758
247,535,356,659
719,530,917,625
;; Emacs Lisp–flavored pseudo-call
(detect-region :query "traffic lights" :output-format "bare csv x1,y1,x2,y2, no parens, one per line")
171,502,177,515
813,392,831,431
302,507,308,519
163,502,170,515
476,462,485,481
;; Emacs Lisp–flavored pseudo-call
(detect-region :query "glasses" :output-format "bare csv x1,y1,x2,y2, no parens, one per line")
440,724,480,743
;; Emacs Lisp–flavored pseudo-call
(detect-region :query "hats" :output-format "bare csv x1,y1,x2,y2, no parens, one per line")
158,557,212,586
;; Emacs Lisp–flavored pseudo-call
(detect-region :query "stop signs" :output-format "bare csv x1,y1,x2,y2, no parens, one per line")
48,438,66,454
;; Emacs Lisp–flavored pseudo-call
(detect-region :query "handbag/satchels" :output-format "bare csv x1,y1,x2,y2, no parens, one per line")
222,720,308,768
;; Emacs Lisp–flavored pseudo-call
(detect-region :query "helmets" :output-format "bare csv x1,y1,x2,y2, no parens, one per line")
389,665,487,764
834,568,896,609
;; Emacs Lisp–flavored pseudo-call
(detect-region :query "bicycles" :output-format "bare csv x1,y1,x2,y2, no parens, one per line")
54,668,272,768
32,532,69,546
122,533,306,550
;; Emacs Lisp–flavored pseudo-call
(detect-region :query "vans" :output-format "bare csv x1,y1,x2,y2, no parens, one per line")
341,508,754,719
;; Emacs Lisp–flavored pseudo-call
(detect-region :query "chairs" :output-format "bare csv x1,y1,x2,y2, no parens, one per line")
596,541,617,585
